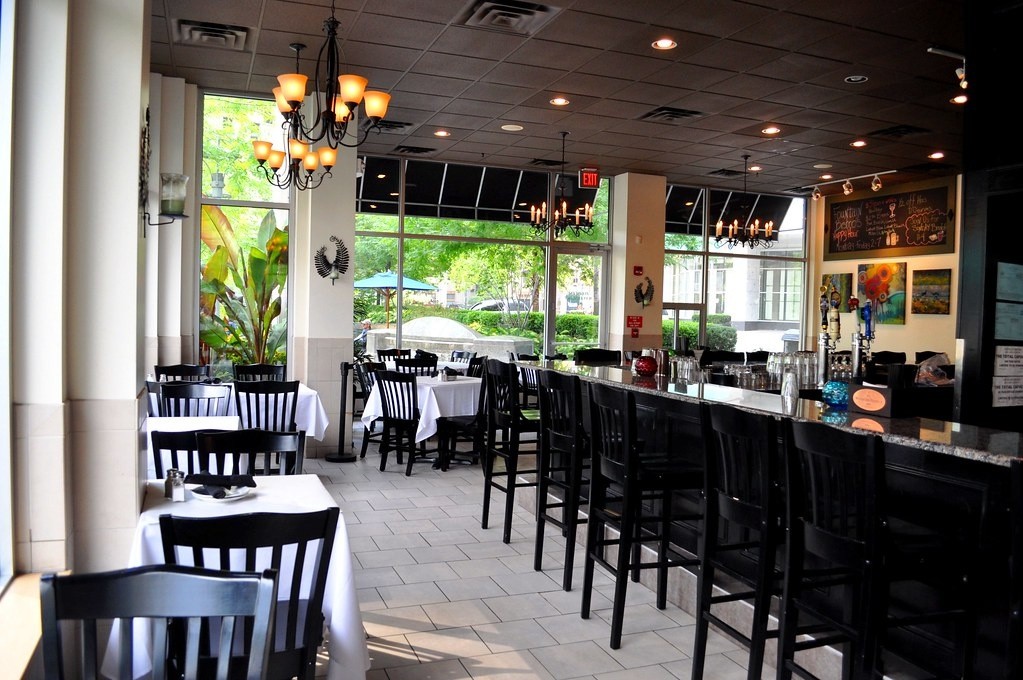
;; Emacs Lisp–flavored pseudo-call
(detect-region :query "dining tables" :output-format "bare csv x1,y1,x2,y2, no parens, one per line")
379,361,469,372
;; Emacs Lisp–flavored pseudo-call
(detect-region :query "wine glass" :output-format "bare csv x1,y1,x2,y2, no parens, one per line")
693,349,704,383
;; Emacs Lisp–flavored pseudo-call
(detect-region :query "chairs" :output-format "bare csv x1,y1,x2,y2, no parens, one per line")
40,365,339,680
358,348,969,680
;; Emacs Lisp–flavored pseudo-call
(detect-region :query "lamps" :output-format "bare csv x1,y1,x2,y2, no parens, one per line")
251,0,390,190
948,64,970,105
527,131,596,237
813,179,883,199
714,155,776,247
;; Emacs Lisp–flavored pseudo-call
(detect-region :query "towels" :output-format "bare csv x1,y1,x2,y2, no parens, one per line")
185,469,256,500
414,348,438,360
554,353,567,360
192,377,221,384
431,366,464,379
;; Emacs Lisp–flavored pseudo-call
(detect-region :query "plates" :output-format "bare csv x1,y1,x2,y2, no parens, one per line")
447,375,457,380
190,485,250,502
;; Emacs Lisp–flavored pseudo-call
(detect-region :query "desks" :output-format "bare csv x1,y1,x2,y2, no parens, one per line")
101,474,371,680
148,381,326,477
363,376,483,466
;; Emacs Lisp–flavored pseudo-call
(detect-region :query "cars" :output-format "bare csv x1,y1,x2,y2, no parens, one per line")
468,299,529,313
449,303,465,310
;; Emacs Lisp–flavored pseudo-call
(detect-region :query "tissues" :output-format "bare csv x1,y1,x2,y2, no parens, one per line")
848,381,912,418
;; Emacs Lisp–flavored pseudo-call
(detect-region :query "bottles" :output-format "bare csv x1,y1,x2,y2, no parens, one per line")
437,369,447,381
677,355,693,382
821,371,851,411
164,470,185,504
766,351,818,385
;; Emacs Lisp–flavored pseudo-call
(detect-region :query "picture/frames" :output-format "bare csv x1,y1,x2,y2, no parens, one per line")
822,263,952,325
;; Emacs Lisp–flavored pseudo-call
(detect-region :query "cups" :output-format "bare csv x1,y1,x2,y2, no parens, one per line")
780,397,797,416
781,372,799,397
